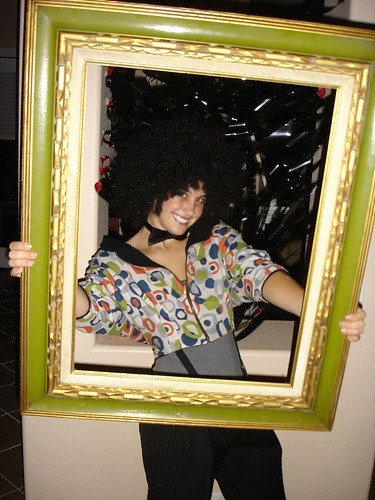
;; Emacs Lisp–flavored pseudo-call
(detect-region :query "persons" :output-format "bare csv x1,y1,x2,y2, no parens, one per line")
8,104,364,500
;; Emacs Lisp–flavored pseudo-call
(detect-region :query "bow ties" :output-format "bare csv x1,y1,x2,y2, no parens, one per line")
144,221,188,246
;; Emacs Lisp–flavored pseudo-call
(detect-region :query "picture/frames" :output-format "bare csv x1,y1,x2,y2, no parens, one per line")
17,0,375,433
